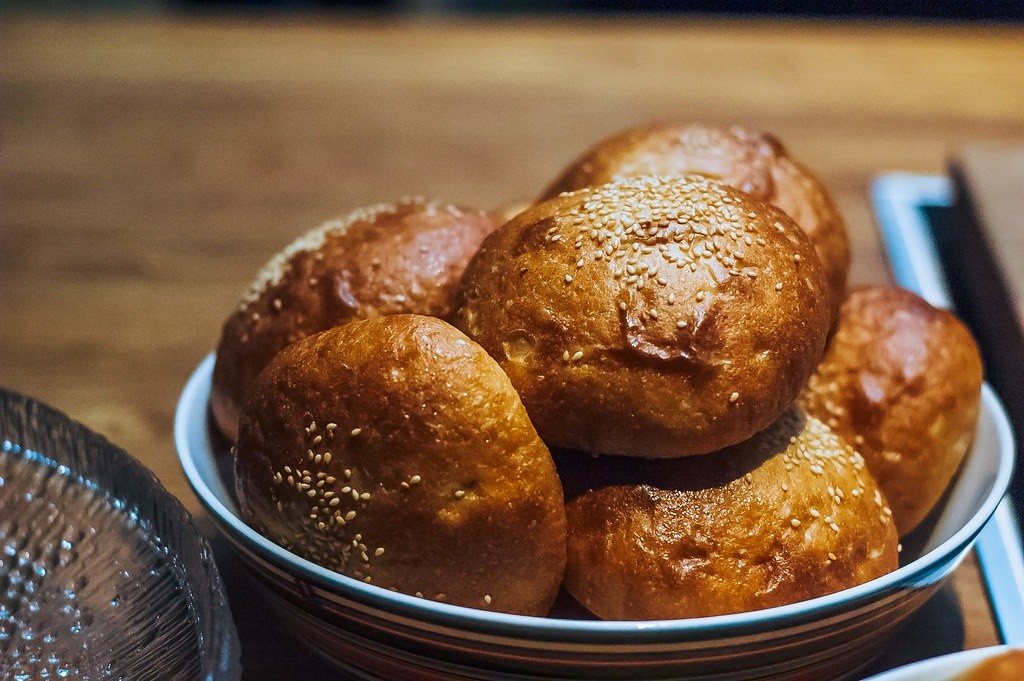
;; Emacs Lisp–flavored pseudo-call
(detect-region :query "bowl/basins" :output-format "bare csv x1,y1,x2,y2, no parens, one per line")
0,383,242,681
173,350,1013,680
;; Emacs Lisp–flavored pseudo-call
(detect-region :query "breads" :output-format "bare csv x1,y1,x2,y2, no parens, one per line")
208,119,984,622
